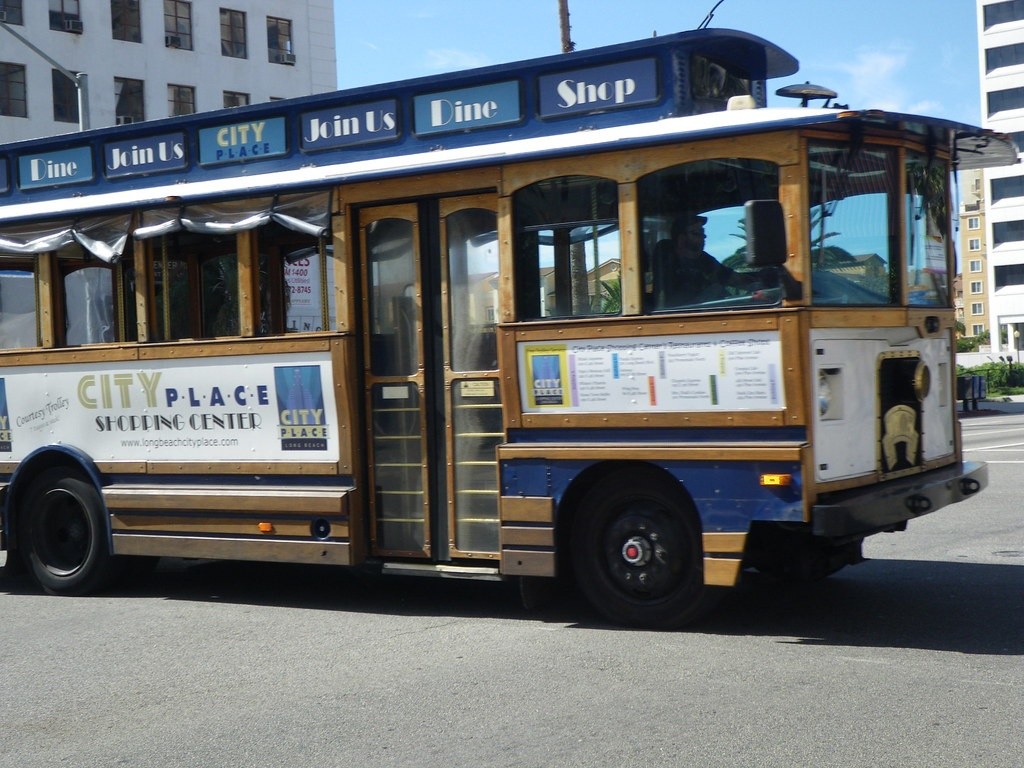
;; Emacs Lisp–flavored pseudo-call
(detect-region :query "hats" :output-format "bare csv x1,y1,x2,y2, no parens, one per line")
670,213,707,241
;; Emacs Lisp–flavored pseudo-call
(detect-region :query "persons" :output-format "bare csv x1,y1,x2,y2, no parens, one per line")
652,211,769,310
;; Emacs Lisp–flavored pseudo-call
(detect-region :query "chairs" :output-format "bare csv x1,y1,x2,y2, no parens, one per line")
653,239,673,310
102,296,115,342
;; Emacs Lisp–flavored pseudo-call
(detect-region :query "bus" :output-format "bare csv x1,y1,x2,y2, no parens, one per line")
0,19,1009,634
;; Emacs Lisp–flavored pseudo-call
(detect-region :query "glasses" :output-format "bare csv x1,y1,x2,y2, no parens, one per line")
688,229,706,239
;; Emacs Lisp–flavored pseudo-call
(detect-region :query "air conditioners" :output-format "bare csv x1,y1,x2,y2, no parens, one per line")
0,11,7,22
117,116,134,125
65,20,83,33
280,54,296,64
165,36,181,48
